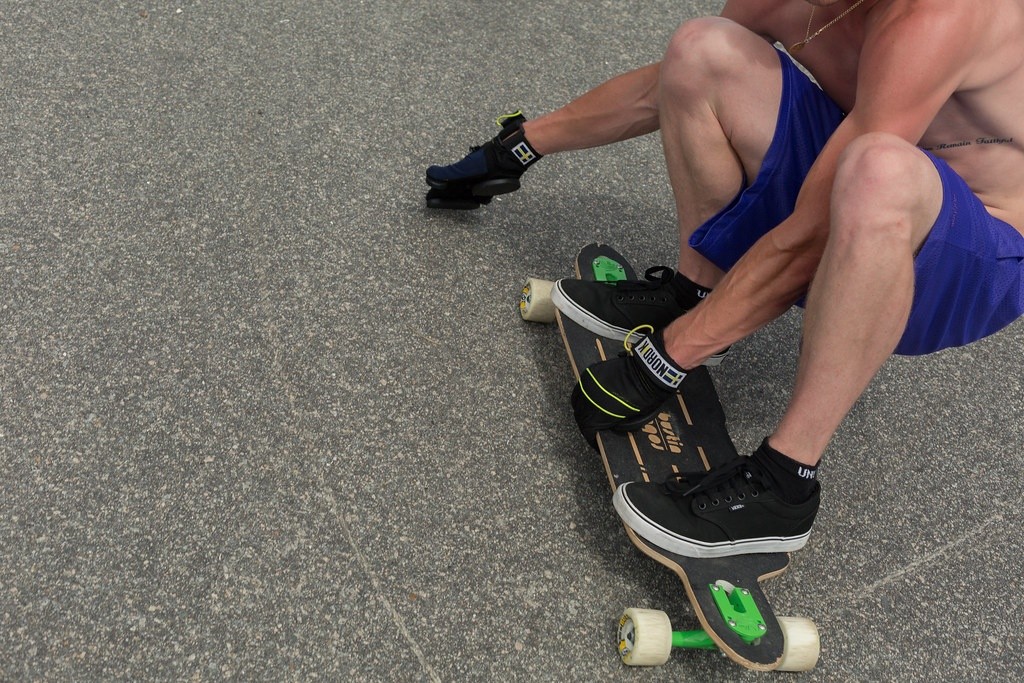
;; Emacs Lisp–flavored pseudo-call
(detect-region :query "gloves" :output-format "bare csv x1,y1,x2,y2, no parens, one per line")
570,327,689,453
425,110,543,210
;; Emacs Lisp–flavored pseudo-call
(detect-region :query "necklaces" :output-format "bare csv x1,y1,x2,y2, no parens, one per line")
790,0,864,55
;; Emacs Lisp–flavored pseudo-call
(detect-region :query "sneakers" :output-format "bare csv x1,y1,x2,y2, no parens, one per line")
550,266,731,367
612,436,821,558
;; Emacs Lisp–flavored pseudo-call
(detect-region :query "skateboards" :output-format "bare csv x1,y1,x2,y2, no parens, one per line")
518,241,821,672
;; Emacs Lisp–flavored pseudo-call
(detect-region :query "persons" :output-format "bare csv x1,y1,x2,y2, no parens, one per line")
425,0,1024,557
